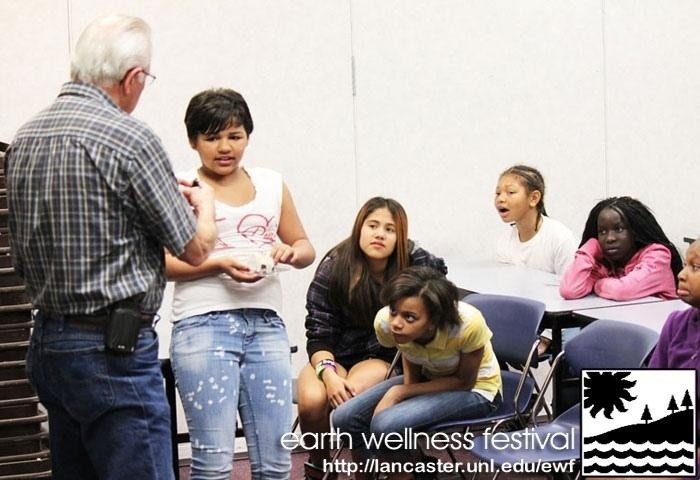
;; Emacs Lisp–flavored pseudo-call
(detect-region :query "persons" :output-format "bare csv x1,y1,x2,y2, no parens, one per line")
646,237,699,368
2,9,218,480
161,83,317,480
331,264,504,480
295,194,449,479
558,195,683,302
492,165,581,357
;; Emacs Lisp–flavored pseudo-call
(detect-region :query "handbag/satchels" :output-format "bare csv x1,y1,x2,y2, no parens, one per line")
105,300,141,354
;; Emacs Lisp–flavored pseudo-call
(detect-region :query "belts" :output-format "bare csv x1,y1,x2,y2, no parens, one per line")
48,312,154,328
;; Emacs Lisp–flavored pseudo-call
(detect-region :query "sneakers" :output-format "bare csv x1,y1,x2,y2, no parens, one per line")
304,455,338,480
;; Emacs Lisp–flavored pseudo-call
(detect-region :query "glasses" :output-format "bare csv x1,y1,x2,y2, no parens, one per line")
120,67,157,86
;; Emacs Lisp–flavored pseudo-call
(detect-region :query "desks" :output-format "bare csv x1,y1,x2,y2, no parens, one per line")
444,236,697,419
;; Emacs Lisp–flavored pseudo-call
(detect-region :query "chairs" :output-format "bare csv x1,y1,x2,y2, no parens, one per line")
159,293,661,480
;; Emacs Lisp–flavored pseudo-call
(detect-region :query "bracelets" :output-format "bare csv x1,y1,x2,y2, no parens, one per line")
314,359,339,379
